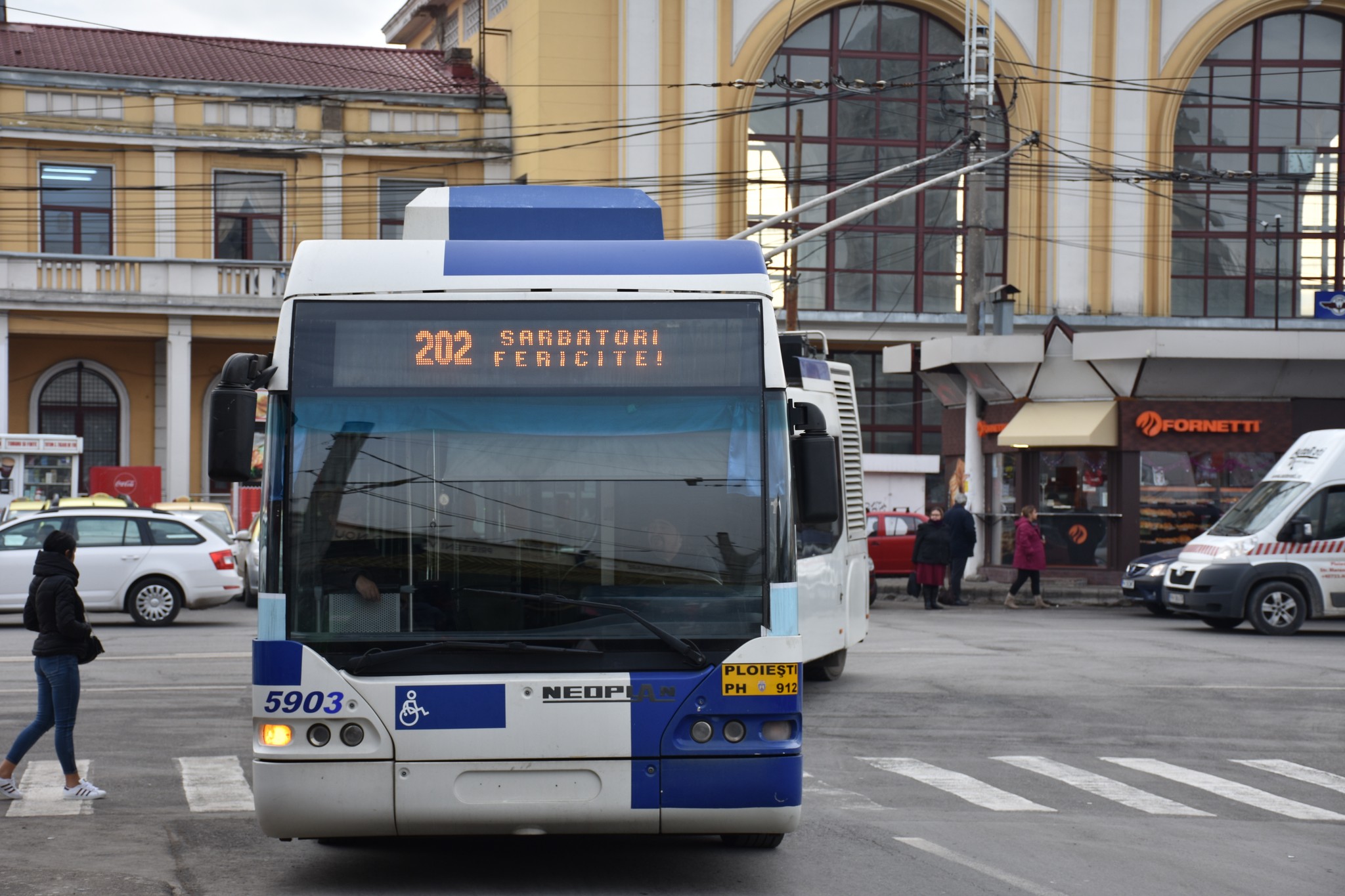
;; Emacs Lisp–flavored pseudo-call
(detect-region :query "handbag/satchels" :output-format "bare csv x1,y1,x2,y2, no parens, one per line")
77,635,105,665
907,564,921,598
938,587,955,605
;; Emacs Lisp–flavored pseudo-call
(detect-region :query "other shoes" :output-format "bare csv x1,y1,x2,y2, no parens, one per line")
955,598,969,605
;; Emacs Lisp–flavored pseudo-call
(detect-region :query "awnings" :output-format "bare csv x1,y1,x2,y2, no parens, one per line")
997,401,1119,448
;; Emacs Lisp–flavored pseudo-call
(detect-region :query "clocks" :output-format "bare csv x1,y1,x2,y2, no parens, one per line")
1281,145,1317,178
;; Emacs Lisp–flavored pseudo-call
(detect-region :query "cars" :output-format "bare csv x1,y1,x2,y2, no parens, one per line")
0,491,262,627
866,506,931,575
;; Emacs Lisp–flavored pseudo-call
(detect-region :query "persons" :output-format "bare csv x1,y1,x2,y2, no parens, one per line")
943,493,976,605
0,530,107,799
912,506,952,610
1004,505,1051,609
321,489,410,602
616,501,723,585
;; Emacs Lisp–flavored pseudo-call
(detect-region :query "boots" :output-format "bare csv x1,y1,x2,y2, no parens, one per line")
923,589,936,610
931,589,944,609
1004,593,1019,609
1034,594,1050,608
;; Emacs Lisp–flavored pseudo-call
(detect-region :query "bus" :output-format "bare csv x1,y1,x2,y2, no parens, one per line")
208,102,1042,852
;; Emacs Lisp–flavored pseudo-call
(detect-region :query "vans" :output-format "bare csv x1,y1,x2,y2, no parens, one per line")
1121,428,1345,635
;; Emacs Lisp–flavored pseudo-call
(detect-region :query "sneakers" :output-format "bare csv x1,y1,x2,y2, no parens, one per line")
63,778,106,799
0,773,22,799
